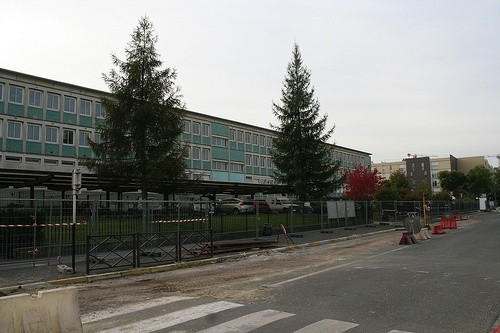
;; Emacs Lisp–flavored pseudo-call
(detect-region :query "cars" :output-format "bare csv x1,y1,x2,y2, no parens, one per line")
214,198,256,215
254,200,272,213
295,202,324,214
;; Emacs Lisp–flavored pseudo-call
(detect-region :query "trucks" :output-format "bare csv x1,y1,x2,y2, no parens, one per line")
264,196,298,213
